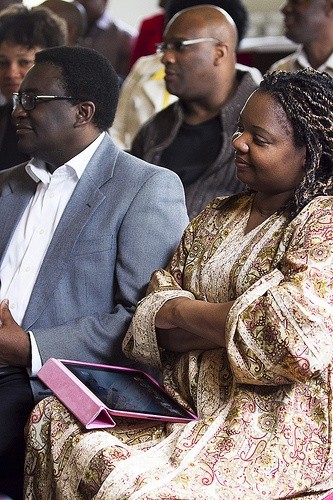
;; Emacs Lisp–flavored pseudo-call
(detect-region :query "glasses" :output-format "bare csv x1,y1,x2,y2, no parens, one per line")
12,93,80,111
156,38,220,56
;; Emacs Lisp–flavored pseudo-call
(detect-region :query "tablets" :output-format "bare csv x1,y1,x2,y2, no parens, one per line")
58,359,198,423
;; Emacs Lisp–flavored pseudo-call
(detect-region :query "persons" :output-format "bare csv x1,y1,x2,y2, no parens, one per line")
123,6,261,223
107,0,265,151
264,0,333,78
0,0,172,91
0,6,68,172
23,67,333,500
0,46,191,500
